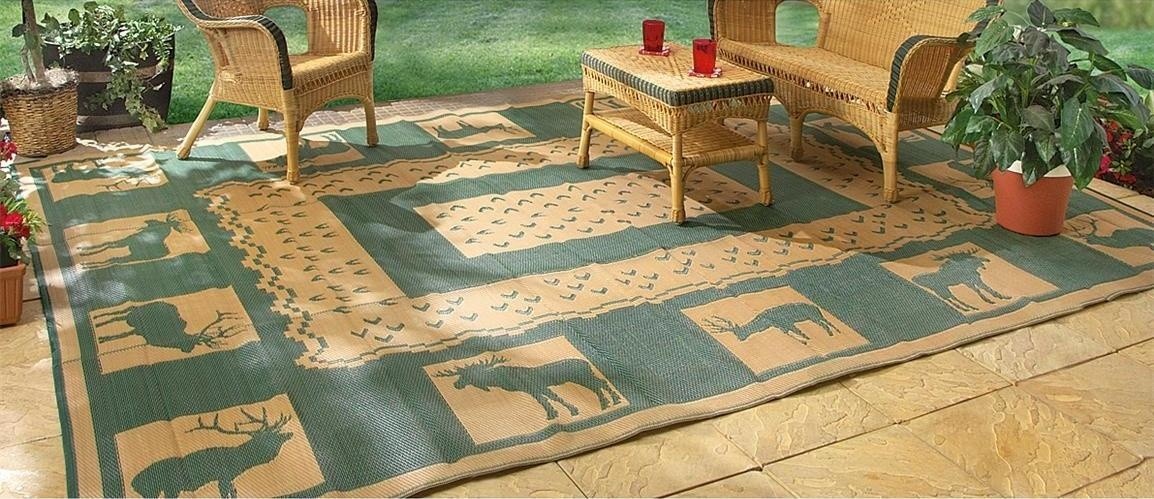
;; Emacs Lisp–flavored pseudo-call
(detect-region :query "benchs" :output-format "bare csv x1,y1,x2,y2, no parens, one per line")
704,0,1003,206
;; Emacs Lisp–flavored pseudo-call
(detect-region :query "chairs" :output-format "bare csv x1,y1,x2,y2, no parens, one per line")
179,0,382,186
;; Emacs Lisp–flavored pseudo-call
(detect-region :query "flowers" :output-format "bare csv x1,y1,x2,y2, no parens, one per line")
0,136,51,269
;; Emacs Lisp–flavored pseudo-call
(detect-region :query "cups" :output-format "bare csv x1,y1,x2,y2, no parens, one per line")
692,38,717,74
642,20,665,52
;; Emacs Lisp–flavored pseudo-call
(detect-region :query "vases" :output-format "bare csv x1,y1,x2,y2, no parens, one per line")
0,265,29,330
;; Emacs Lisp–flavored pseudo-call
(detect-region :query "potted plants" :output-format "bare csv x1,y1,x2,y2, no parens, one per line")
5,2,182,135
941,1,1154,239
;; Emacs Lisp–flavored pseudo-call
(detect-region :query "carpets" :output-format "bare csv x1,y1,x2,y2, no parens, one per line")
10,93,1154,498
